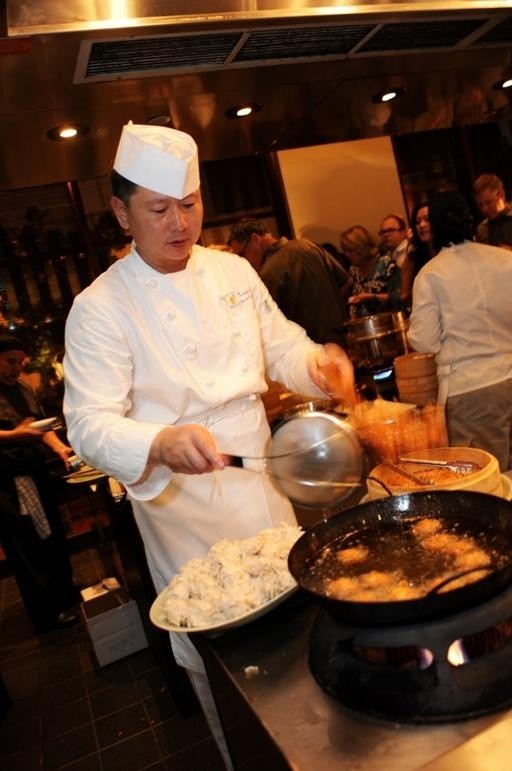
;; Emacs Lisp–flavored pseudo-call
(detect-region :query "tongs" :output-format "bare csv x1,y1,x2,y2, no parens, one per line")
377,453,483,489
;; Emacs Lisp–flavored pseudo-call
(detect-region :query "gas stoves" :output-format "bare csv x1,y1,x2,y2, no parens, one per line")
305,589,510,722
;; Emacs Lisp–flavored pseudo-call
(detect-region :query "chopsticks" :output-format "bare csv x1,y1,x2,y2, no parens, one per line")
63,466,104,480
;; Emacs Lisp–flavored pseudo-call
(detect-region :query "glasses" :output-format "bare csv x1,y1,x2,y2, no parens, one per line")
238,239,250,256
378,228,401,235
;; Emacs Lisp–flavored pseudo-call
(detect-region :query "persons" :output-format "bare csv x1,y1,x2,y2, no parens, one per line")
404,187,512,474
94,209,131,260
2,338,87,635
228,199,432,347
57,119,364,770
473,173,511,249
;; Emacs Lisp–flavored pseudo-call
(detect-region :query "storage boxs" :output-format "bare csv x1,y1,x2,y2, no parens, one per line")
79,596,149,668
81,577,122,618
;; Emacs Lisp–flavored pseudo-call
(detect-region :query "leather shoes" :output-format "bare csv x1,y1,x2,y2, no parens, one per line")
52,611,79,626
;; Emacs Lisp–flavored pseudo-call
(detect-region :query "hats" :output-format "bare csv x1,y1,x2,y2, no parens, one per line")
113,124,201,199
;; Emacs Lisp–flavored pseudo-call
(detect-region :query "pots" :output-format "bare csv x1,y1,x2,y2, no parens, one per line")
285,477,512,628
338,310,412,375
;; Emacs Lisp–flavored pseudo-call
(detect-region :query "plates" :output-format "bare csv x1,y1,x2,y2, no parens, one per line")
147,530,304,634
67,465,108,484
26,417,59,434
